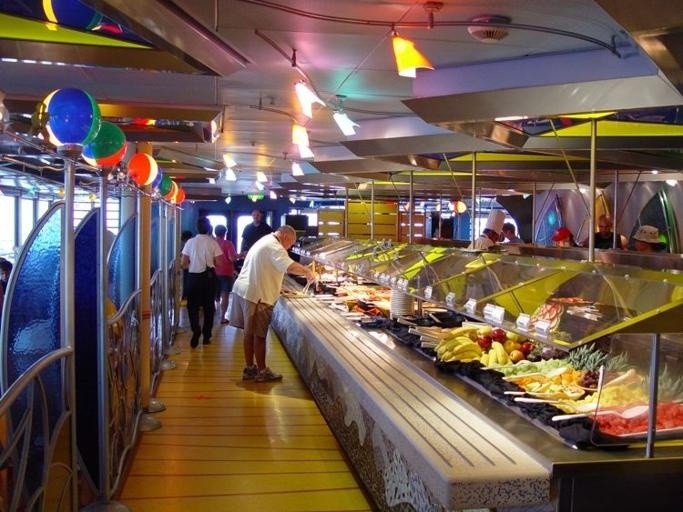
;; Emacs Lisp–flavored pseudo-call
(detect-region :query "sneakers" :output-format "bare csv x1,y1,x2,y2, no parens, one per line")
190,319,229,349
243,365,282,383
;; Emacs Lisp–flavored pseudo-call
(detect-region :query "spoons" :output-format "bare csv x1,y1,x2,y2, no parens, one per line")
513,396,596,412
552,404,649,422
500,367,566,380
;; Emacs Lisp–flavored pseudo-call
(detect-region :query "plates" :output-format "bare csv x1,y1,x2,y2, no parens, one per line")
389,288,415,321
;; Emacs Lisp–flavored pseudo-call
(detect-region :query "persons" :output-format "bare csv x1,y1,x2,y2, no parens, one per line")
241,208,273,254
229,225,316,383
503,223,525,243
179,218,244,349
0,262,12,294
583,215,628,251
627,224,675,253
467,228,499,250
552,227,585,260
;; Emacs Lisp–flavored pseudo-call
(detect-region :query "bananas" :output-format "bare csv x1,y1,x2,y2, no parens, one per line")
435,324,512,373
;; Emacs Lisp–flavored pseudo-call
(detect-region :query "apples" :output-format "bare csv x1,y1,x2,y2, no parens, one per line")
477,326,533,363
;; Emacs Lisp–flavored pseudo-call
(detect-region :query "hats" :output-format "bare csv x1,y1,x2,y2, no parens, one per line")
633,224,660,244
550,227,571,242
484,209,506,236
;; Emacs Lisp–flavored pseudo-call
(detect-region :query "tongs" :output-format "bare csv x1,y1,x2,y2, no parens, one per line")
302,280,311,295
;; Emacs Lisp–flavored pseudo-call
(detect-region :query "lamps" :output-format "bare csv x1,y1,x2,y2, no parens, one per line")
222,19,440,200
